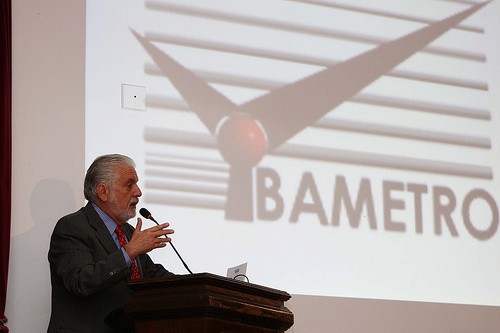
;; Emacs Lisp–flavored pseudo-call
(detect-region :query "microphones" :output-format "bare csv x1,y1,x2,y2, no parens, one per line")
140,208,193,274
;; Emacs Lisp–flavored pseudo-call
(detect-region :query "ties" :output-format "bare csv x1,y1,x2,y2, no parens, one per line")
115,225,142,281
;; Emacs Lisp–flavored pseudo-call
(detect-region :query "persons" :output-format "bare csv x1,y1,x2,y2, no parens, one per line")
48,153,183,333
